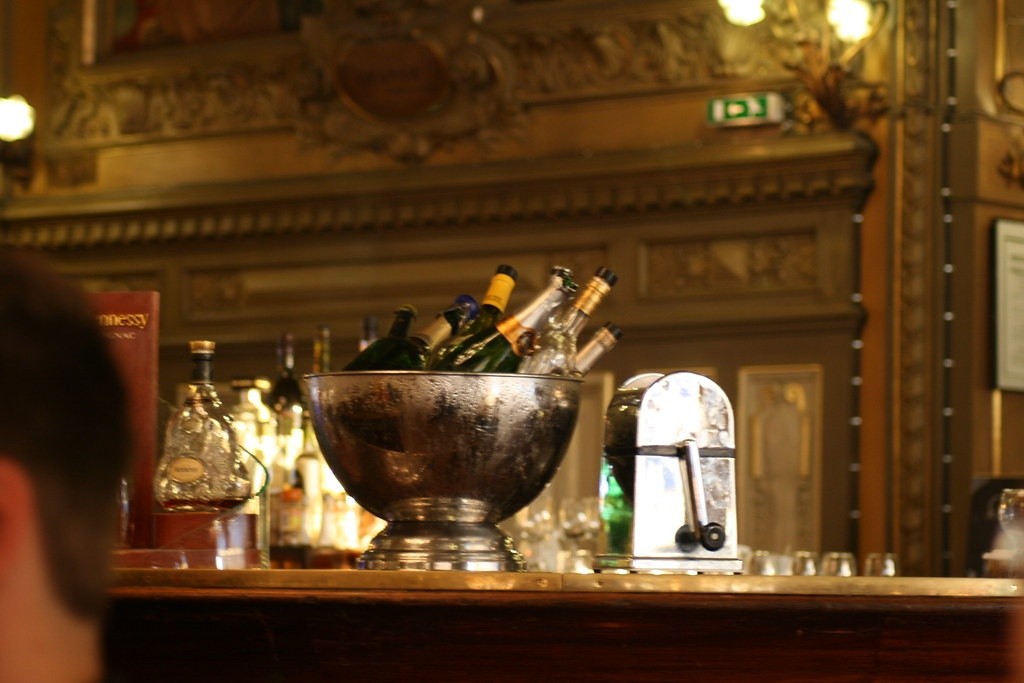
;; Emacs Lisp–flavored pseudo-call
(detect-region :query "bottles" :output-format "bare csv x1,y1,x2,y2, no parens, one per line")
152,339,254,513
268,326,335,439
340,261,623,380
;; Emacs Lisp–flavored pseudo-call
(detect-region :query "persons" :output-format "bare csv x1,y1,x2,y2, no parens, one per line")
0,255,130,683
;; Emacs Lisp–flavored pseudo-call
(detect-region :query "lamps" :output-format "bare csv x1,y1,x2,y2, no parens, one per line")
716,0,889,134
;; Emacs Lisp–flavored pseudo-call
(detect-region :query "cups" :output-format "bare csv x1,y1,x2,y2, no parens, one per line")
736,543,903,579
